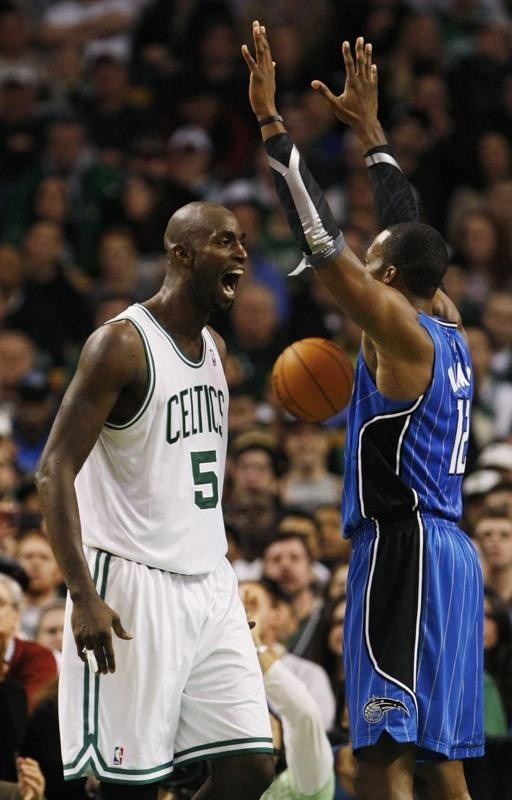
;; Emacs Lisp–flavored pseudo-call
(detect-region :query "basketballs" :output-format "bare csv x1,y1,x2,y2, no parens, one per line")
273,336,356,426
